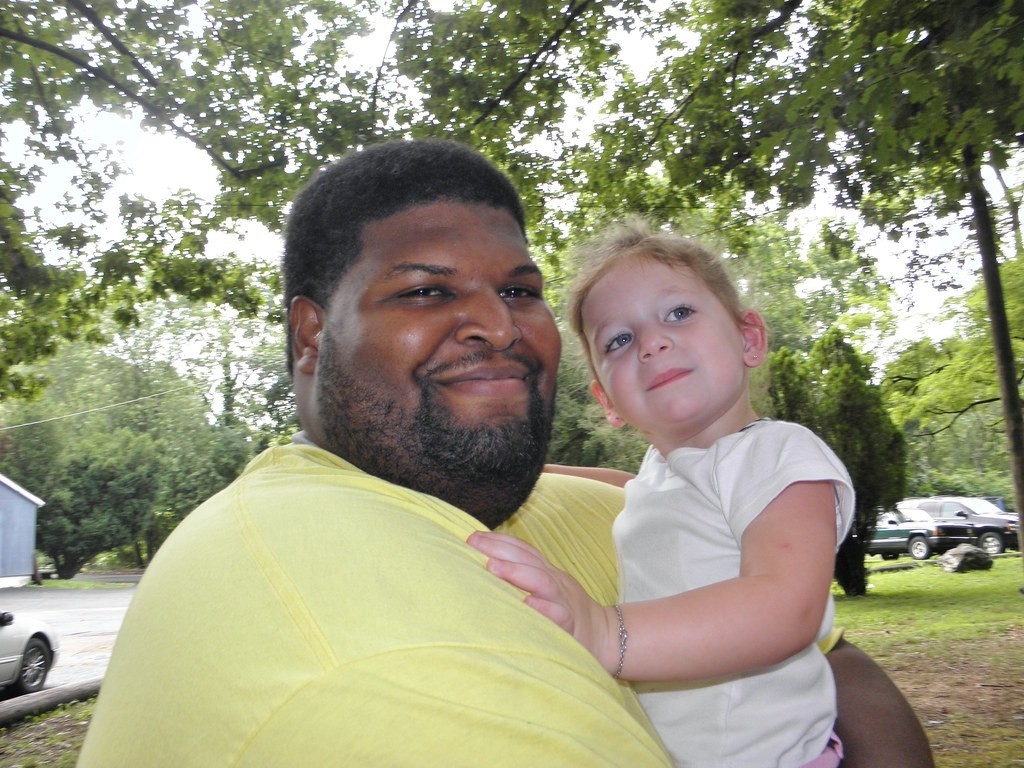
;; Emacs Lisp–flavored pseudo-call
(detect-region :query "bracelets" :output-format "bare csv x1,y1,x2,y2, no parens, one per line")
611,604,627,680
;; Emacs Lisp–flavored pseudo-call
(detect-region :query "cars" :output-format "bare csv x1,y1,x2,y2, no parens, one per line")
892,496,1021,557
864,505,980,561
0,607,61,699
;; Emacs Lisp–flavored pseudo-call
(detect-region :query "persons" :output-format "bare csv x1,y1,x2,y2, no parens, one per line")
73,138,935,768
468,214,855,768
